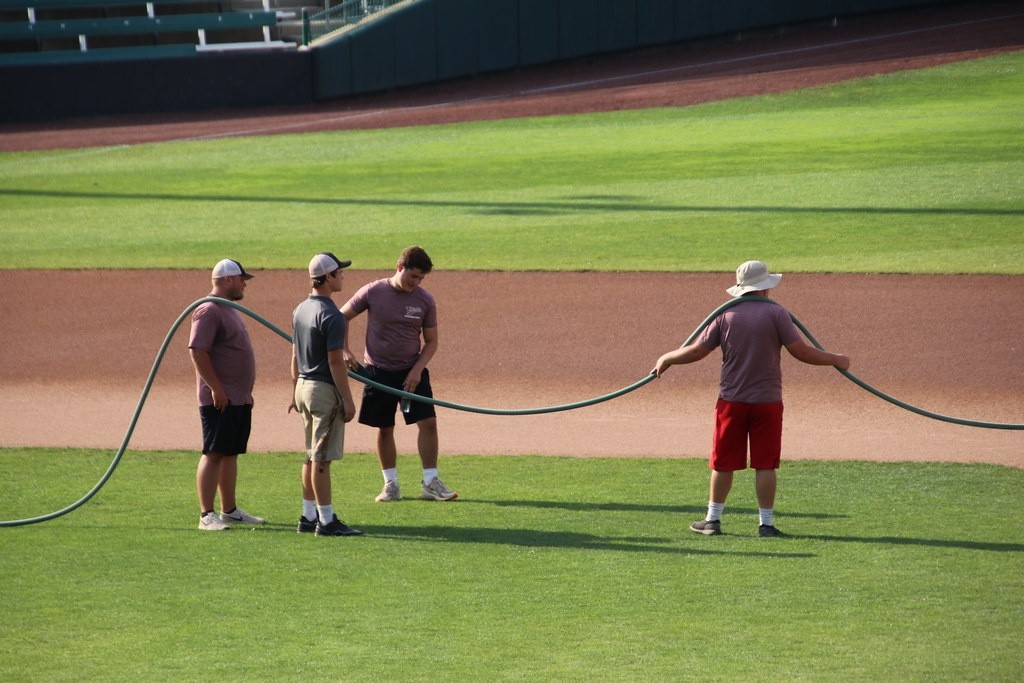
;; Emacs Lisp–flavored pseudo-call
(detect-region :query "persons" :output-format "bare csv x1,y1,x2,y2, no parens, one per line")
188,258,267,530
339,245,458,502
650,260,851,538
288,252,363,535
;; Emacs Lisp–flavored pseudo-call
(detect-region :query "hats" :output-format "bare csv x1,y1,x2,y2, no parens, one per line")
211,258,256,280
725,260,783,298
308,252,352,279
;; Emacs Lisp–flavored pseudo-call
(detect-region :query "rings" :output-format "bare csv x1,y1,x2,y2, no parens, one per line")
349,364,352,368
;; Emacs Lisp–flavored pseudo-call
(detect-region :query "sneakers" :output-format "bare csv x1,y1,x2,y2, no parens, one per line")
689,519,723,535
198,511,230,532
219,507,268,524
757,524,781,536
295,509,319,533
375,480,401,503
314,513,365,536
420,475,458,500
